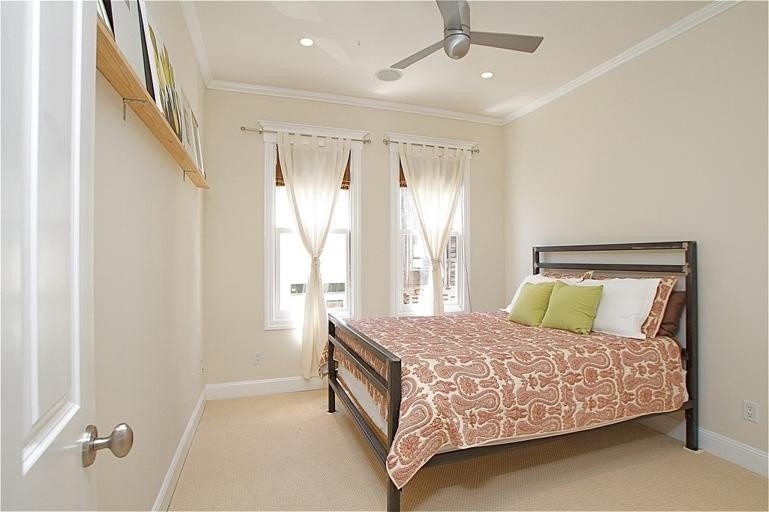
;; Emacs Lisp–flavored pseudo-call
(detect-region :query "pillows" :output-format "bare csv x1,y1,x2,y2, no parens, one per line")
499,271,687,340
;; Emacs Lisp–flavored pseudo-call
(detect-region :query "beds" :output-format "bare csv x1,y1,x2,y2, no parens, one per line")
328,241,697,512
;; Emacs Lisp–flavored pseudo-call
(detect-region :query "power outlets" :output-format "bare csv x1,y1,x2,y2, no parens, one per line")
743,401,759,423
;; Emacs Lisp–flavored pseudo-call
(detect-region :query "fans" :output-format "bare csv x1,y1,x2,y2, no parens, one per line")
390,0,543,69
